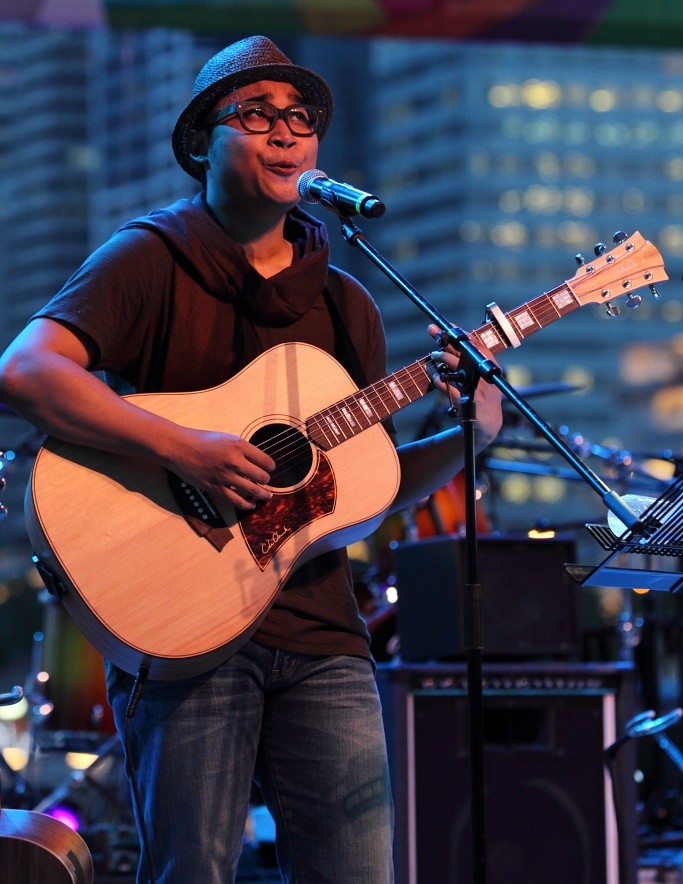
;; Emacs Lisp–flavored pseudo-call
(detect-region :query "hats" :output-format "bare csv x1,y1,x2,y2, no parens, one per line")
172,34,334,185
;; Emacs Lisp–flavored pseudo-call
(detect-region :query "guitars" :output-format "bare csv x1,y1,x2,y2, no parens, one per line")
20,225,674,683
0,808,97,884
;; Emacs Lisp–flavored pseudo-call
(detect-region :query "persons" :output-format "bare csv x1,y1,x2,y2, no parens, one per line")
1,36,504,883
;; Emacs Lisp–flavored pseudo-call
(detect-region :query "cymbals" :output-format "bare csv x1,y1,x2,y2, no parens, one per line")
398,382,585,431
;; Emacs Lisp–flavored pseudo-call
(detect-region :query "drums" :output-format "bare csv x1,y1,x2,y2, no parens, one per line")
370,468,498,567
34,731,126,821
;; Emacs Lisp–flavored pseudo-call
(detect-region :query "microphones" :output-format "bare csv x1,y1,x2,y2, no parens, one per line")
297,169,386,219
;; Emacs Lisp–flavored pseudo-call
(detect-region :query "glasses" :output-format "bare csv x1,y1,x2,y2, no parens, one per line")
203,100,325,137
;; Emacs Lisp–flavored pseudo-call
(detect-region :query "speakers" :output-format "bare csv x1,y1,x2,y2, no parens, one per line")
373,660,636,884
394,536,576,661
